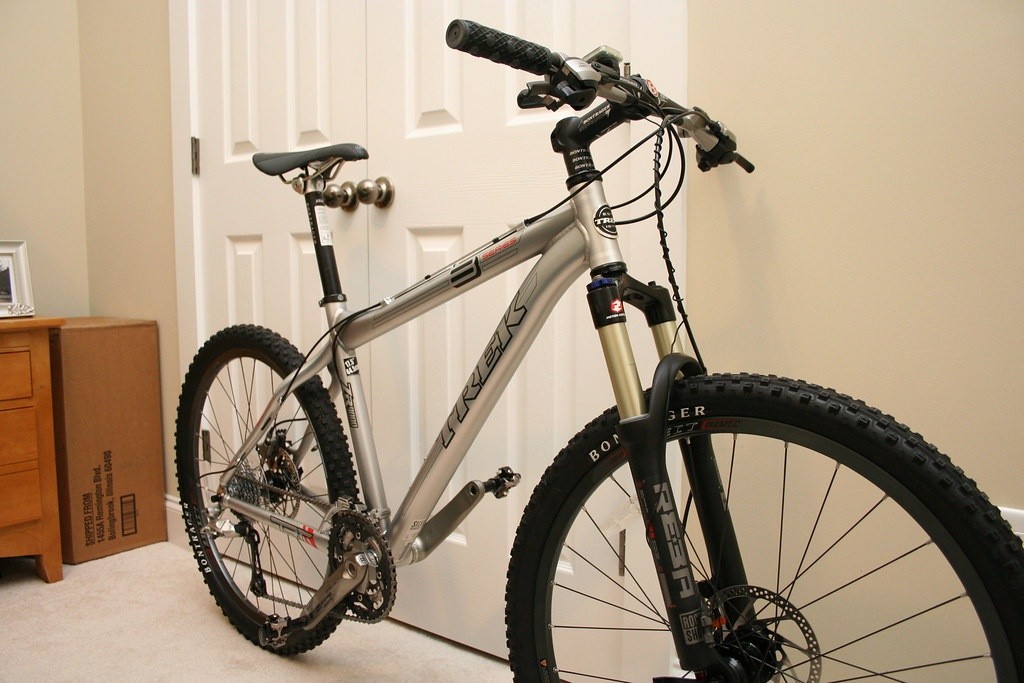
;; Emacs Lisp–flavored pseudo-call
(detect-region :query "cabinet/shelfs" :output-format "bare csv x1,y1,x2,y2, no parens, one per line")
0,316,64,586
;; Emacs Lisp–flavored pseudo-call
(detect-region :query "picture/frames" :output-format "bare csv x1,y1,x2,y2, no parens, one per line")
0,240,36,318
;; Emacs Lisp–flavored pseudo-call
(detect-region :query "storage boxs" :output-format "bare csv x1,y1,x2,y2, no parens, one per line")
51,315,170,566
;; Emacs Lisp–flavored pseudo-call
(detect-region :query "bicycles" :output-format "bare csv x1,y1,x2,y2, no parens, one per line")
172,15,1023,682
